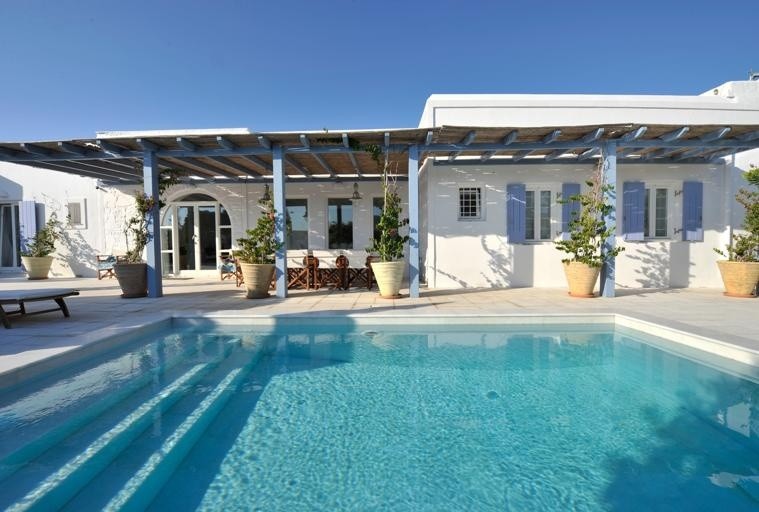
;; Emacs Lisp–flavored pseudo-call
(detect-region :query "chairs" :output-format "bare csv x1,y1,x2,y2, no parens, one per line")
216,255,237,281
96,254,115,279
0,288,80,330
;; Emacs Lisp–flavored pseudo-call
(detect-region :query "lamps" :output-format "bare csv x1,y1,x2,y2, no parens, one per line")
258,168,273,203
348,168,360,202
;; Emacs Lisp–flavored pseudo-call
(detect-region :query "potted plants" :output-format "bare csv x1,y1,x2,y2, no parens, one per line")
553,181,625,298
713,165,759,297
112,192,156,299
232,192,286,299
17,211,72,279
366,162,410,299
